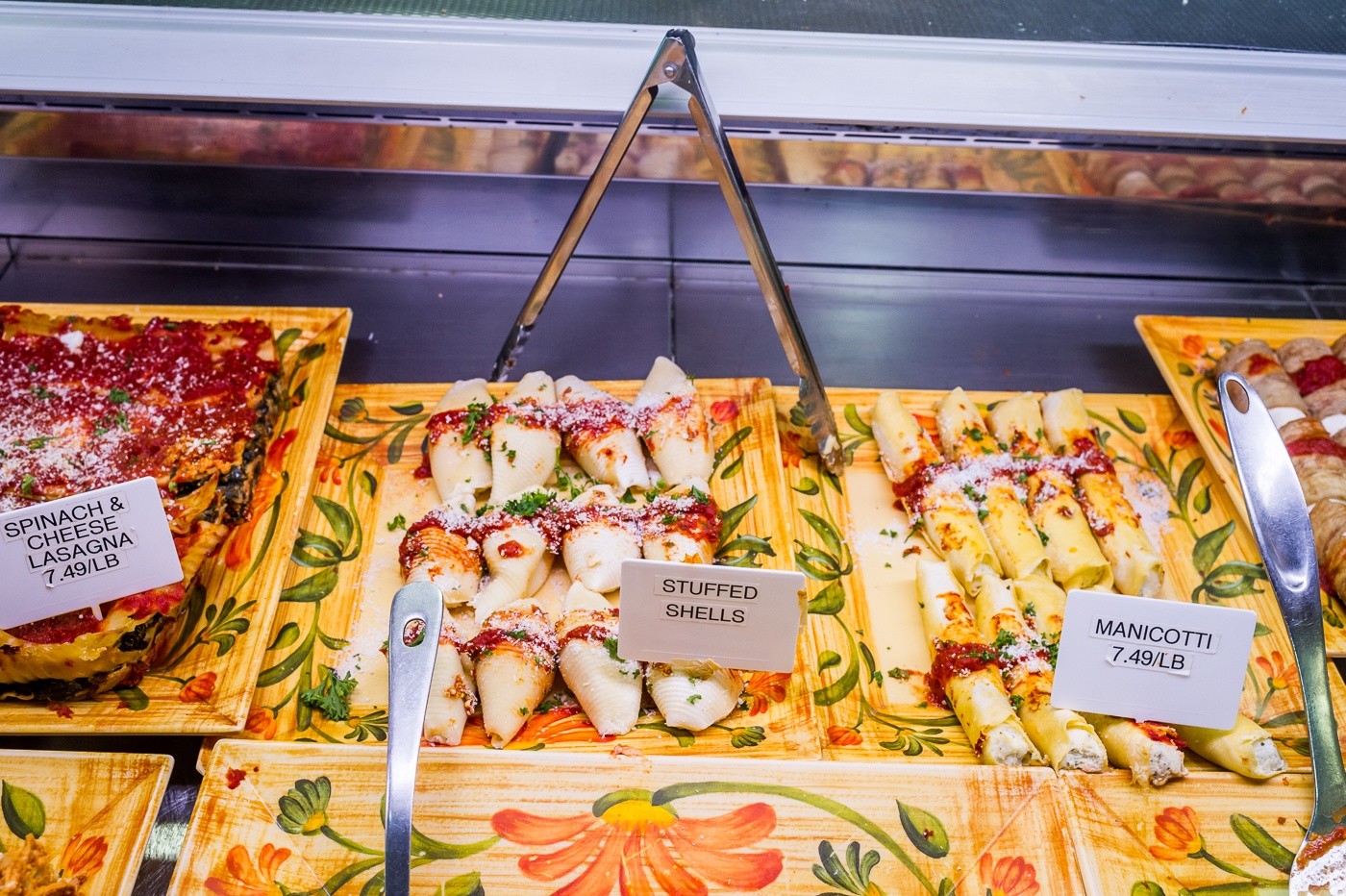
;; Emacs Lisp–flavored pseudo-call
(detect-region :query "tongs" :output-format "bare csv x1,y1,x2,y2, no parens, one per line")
487,30,849,477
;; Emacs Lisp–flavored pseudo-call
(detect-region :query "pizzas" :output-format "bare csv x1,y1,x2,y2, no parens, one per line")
0,304,287,705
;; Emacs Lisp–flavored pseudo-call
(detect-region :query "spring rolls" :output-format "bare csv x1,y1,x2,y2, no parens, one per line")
874,385,1288,787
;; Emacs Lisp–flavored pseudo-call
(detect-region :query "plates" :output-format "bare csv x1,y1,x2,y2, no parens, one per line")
1133,313,1345,657
0,749,175,896
194,378,823,775
1057,767,1345,896
0,301,354,735
166,736,1085,896
775,385,1346,772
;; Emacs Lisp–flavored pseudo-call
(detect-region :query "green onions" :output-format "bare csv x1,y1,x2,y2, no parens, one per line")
386,390,1066,712
